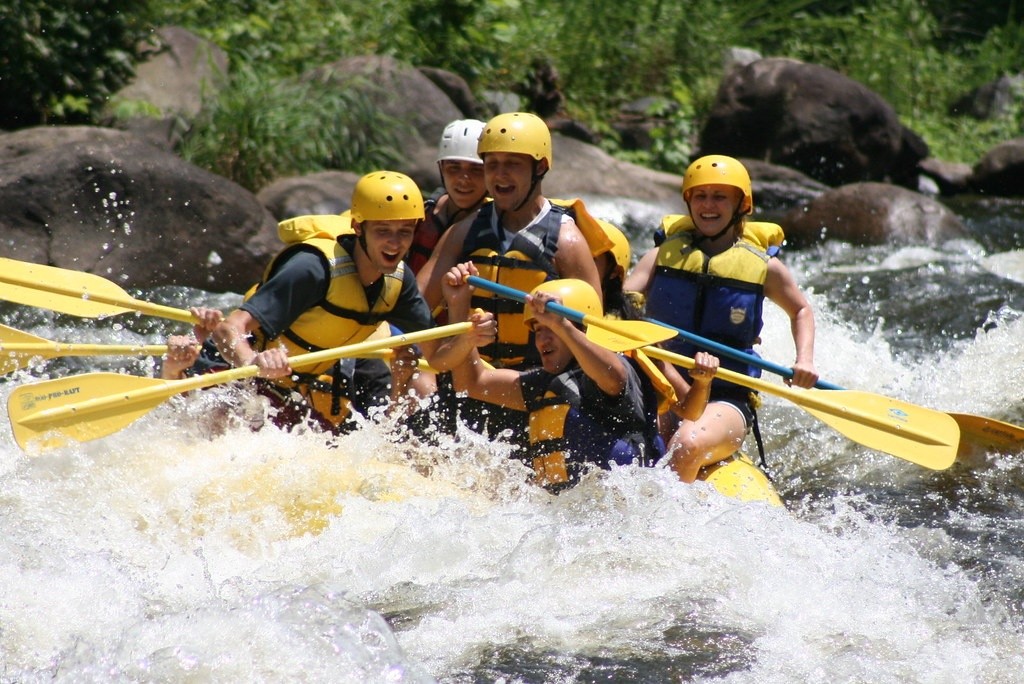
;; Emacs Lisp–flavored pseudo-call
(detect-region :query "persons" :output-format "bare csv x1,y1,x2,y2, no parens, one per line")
623,153,819,484
164,112,719,496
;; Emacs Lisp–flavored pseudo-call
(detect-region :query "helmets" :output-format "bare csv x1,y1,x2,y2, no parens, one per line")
436,119,487,164
244,283,258,302
523,278,603,331
682,155,753,216
477,112,552,171
351,171,426,221
595,217,631,281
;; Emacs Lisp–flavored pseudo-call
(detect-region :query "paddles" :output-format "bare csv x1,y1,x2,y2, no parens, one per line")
449,271,680,354
1,257,224,330
1,325,204,376
7,308,483,456
623,291,1023,462
640,344,961,470
